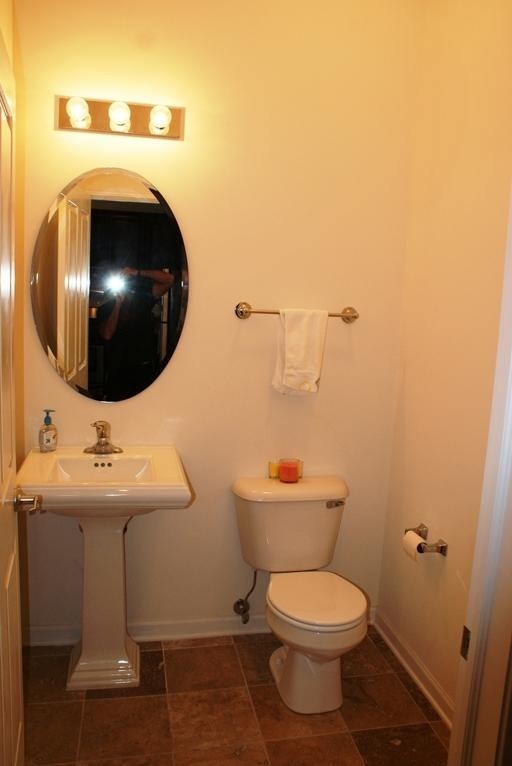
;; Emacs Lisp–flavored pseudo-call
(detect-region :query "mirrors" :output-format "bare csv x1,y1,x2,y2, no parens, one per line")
28,168,187,401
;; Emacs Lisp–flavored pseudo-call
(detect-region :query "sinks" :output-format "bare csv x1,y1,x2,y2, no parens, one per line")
15,445,192,521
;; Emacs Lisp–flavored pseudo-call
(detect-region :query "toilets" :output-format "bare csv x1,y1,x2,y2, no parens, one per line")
233,476,369,714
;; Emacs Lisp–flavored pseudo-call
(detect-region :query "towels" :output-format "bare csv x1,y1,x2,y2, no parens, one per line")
270,307,329,395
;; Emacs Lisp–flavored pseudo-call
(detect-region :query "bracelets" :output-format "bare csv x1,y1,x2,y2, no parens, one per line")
135,268,141,278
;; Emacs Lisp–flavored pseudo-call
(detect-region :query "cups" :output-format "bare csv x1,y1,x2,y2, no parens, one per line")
267,458,304,484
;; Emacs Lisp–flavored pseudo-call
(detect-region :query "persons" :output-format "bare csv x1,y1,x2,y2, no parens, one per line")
98,266,175,401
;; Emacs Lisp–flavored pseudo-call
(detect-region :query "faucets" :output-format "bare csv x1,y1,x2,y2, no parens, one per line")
84,420,123,455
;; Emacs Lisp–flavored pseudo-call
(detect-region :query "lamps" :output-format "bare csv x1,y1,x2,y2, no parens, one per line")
54,94,185,140
